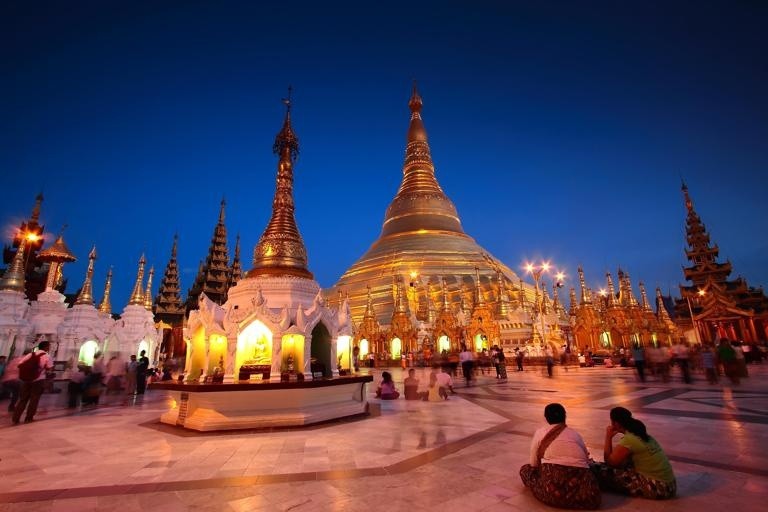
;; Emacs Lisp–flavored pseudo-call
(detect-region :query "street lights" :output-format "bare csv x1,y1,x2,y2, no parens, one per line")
599,287,608,321
526,261,552,355
684,288,709,349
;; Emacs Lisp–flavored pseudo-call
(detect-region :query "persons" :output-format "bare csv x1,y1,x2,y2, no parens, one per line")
0,341,173,424
597,406,678,501
518,402,601,508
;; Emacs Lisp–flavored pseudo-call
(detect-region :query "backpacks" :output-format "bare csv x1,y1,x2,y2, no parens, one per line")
17,352,47,382
